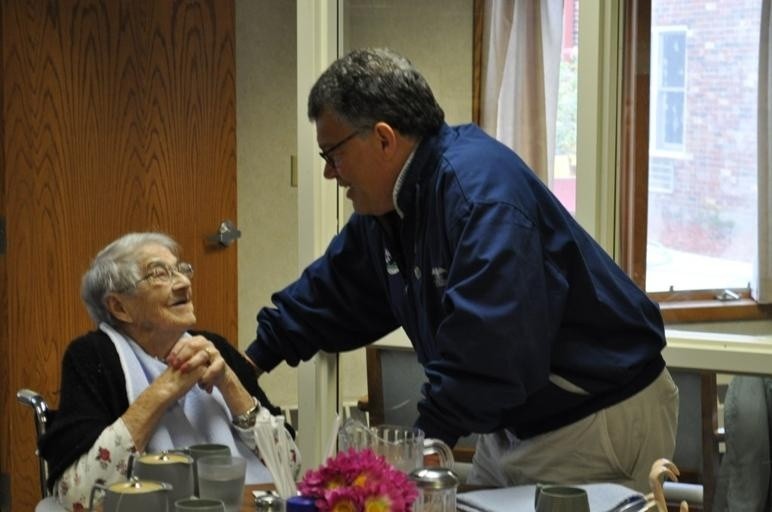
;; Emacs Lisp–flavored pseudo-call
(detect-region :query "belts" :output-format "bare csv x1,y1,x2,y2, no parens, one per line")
510,357,665,440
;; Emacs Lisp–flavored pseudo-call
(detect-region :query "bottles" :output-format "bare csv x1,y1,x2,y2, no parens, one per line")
409,467,460,512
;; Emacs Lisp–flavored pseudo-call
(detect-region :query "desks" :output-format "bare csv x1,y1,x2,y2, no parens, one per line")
69,454,709,512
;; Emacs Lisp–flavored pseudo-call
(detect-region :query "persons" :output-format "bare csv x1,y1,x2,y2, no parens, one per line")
241,47,680,489
38,231,297,512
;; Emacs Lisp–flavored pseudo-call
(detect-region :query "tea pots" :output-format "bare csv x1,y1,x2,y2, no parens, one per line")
89,444,193,512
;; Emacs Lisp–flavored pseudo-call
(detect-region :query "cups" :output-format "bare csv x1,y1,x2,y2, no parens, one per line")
175,443,247,511
534,485,590,511
367,427,455,477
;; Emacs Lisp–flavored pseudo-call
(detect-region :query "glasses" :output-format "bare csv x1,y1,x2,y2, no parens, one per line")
319,130,358,167
119,261,195,295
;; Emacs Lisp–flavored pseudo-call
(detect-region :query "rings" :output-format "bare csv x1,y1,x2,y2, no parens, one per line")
204,347,212,355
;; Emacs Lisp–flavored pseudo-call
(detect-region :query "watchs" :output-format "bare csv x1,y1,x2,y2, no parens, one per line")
232,395,261,429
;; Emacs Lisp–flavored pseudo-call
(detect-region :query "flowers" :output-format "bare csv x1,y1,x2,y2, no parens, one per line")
294,447,422,512
